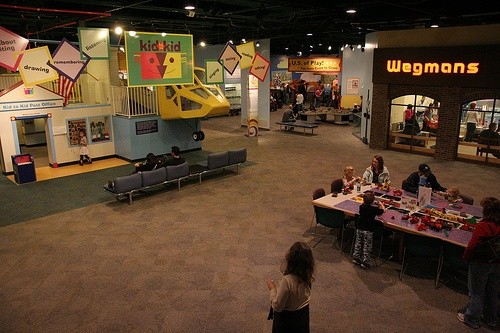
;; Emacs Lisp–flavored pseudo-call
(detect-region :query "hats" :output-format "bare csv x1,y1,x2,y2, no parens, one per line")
419,164,431,172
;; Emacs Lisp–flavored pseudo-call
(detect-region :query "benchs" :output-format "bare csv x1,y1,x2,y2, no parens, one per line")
103,148,247,205
276,122,318,136
389,132,500,150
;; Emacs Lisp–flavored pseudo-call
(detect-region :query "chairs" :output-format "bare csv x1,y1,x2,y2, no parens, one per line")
331,179,343,193
458,193,474,206
313,188,344,248
477,135,500,158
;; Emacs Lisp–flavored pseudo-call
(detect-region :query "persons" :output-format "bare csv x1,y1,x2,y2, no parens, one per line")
92,123,105,139
131,153,159,176
404,102,439,134
457,197,500,329
158,146,185,168
404,164,447,194
270,79,341,112
282,104,296,130
363,155,390,184
342,166,361,189
352,192,385,270
78,130,92,165
480,123,500,160
465,103,478,142
267,243,317,333
353,95,363,127
435,186,464,203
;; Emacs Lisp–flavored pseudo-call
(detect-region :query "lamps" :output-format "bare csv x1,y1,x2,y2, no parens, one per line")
255,34,260,47
285,41,365,56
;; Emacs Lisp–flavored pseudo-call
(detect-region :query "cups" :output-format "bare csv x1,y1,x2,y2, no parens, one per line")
371,183,375,188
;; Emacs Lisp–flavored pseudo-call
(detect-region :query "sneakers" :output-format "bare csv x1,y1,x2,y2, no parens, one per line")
361,262,371,269
457,312,480,328
478,311,497,327
352,258,360,265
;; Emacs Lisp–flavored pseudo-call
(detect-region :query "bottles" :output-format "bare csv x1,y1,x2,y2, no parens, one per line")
357,181,361,192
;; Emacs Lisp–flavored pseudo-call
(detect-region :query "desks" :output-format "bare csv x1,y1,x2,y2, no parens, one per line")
353,113,362,126
298,108,353,124
311,183,484,263
